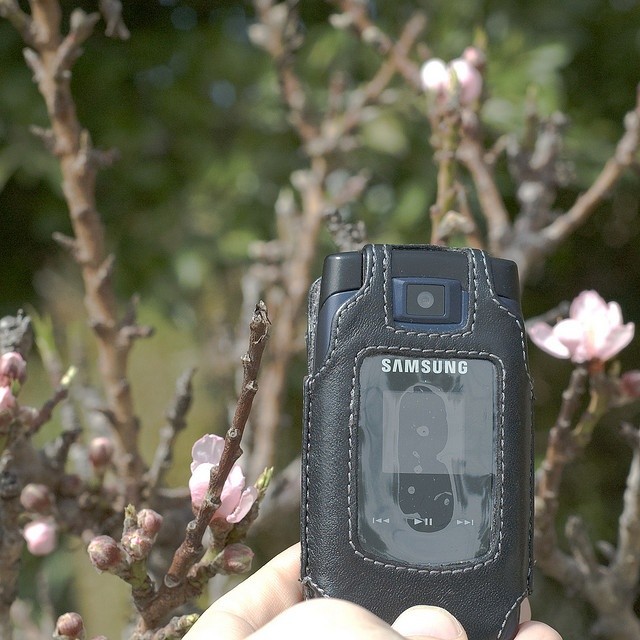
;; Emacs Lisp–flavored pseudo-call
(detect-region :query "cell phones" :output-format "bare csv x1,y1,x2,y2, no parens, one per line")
305,244,533,640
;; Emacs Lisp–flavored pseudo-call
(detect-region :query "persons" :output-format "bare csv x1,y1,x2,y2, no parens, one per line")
182,539,564,640
